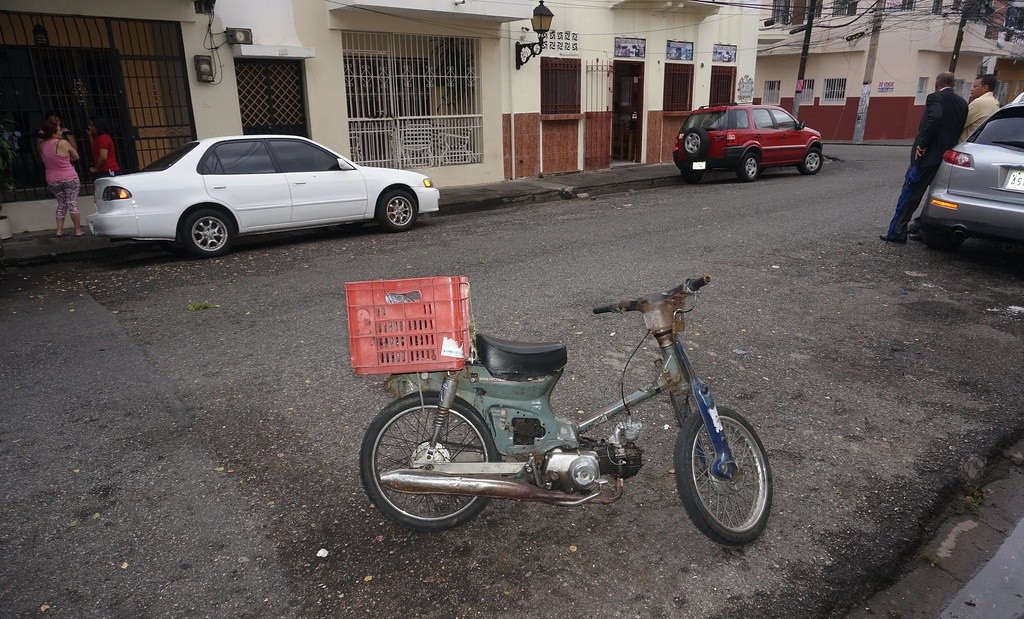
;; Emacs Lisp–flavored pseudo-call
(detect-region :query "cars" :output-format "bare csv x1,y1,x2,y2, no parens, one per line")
86,134,441,257
916,91,1024,250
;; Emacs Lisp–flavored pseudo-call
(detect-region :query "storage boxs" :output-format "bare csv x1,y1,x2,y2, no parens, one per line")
345,274,472,376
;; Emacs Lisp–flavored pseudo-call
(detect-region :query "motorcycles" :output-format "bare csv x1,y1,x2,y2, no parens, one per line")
356,273,775,544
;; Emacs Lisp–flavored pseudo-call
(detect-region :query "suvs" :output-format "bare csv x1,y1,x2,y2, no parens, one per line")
673,102,824,183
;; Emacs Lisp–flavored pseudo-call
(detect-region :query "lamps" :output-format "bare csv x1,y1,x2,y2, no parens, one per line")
515,0,555,70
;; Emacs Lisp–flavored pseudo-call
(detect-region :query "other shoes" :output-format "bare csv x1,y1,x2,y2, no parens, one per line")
879,233,906,242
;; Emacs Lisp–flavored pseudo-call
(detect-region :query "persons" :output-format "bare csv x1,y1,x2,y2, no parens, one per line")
958,74,1000,144
86,118,121,182
879,71,969,244
36,123,85,237
44,112,78,150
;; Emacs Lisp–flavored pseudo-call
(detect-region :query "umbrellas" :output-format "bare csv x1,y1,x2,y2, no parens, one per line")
885,147,925,244
